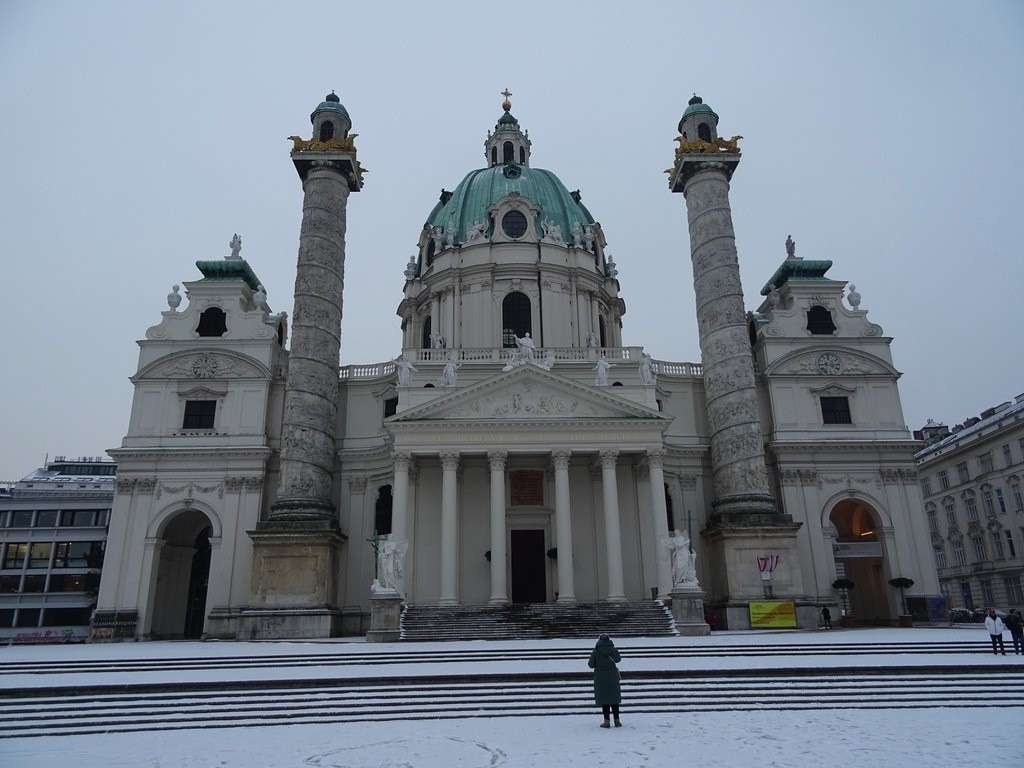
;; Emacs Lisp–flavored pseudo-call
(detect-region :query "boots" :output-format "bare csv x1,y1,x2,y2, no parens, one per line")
600,718,610,728
615,719,622,726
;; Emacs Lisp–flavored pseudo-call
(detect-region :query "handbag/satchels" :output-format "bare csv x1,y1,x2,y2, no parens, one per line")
616,667,622,680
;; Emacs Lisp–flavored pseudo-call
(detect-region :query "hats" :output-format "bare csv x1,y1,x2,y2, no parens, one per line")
987,608,995,613
597,634,610,640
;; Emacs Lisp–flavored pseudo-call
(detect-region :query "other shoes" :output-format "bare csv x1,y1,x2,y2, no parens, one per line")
994,652,997,655
1002,653,1006,655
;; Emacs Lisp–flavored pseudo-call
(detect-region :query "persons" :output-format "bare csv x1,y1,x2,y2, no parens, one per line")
1005,609,1024,655
639,354,652,385
822,606,832,629
378,534,400,589
786,236,794,255
592,356,616,383
232,234,241,255
443,357,462,385
984,608,1006,656
588,634,622,727
950,609,954,624
512,332,536,365
586,333,598,347
468,219,486,240
543,215,561,243
429,332,443,349
671,529,691,582
391,357,419,385
